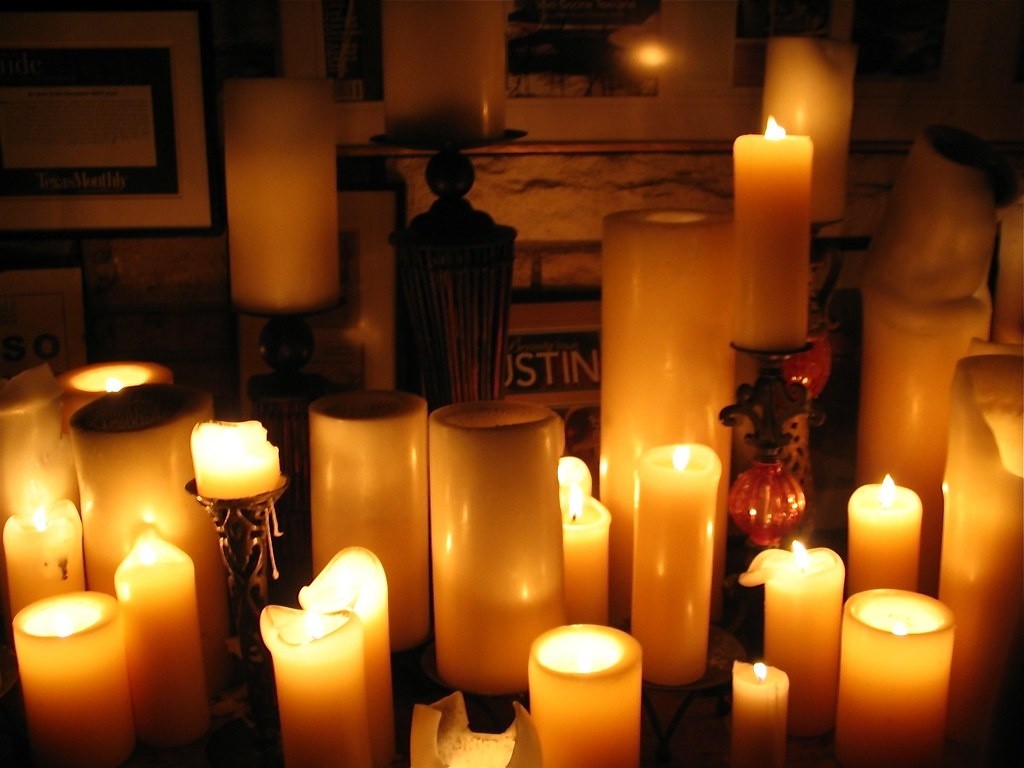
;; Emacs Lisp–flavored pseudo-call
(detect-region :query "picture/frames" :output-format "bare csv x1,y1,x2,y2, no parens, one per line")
212,0,961,107
227,177,405,422
0,246,97,382
420,299,602,500
0,0,227,241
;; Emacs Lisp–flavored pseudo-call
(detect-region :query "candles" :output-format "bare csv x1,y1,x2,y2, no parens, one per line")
0,38,1024,768
